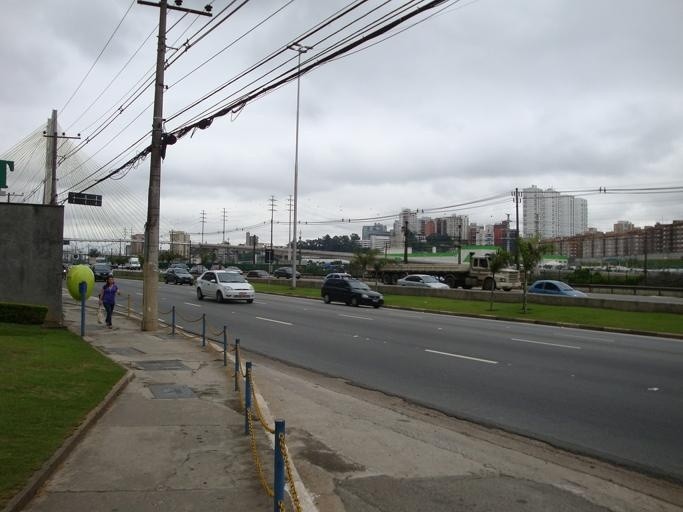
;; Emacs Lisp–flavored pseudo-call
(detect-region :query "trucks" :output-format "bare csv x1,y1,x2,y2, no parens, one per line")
96,258,107,265
129,258,141,270
366,255,522,290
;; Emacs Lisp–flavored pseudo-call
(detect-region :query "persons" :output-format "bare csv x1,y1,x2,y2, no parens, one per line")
62,265,66,280
97,274,120,331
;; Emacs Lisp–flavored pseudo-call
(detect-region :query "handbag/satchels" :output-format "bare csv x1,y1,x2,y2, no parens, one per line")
98,294,104,300
97,312,104,324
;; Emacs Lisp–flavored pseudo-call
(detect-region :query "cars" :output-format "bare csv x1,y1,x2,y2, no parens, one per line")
163,267,194,287
195,268,256,304
110,264,129,269
173,263,244,274
527,279,588,296
324,272,358,279
273,266,300,279
247,269,275,278
92,265,114,282
321,278,384,308
396,274,449,290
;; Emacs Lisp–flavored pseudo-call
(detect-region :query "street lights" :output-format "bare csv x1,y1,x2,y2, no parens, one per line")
284,40,315,288
